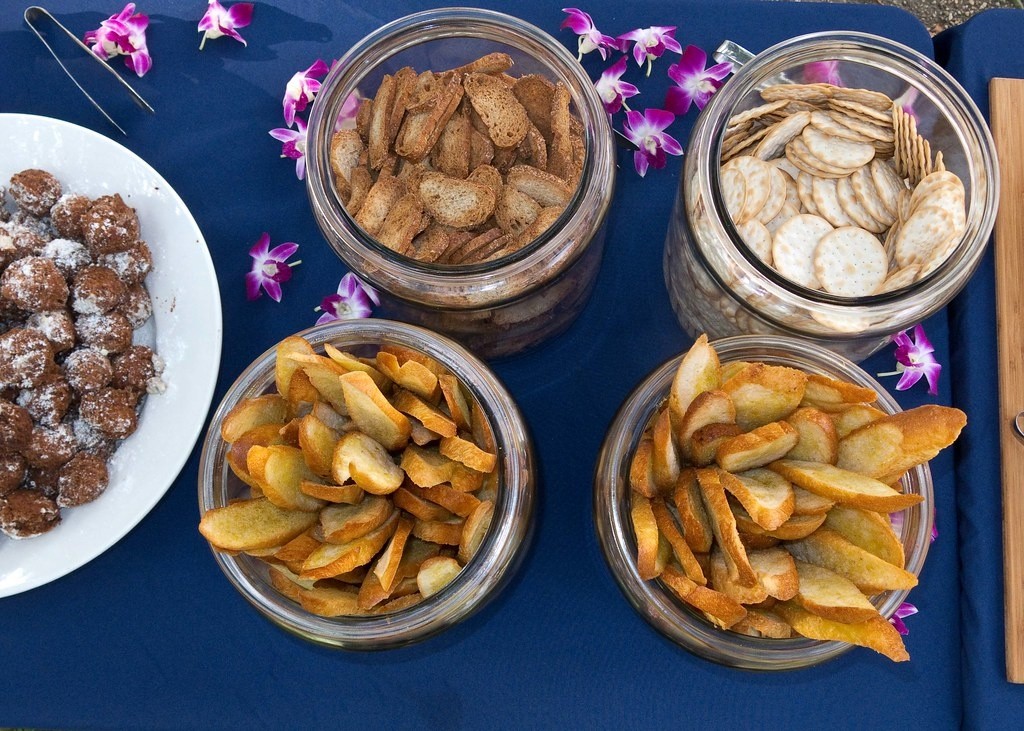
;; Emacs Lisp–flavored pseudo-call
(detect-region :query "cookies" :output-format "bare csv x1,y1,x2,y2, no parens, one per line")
669,81,968,340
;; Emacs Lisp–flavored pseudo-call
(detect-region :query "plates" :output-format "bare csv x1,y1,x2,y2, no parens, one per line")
0,113,223,599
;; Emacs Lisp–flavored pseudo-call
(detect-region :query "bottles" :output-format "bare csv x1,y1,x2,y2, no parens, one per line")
663,31,1001,366
305,6,617,363
198,318,537,651
596,335,934,670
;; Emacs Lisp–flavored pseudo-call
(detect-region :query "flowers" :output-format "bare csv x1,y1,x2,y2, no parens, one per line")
561,7,619,63
246,233,302,302
283,59,329,128
889,504,938,546
664,45,735,115
891,83,920,123
83,2,152,78
197,0,253,51
615,27,683,77
889,602,918,636
802,60,844,88
614,100,685,176
268,116,308,181
329,59,368,131
593,56,641,120
877,324,941,395
314,273,371,327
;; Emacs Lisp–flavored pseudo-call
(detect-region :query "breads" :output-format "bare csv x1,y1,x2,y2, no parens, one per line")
328,52,589,354
630,332,968,661
198,335,501,618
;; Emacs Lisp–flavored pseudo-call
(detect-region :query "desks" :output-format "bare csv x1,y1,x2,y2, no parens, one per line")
933,9,1024,731
0,0,963,731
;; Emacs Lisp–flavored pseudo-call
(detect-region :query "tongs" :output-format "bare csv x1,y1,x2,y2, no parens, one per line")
24,6,155,139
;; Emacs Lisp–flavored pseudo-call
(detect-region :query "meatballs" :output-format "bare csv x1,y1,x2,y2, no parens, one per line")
0,168,163,538
